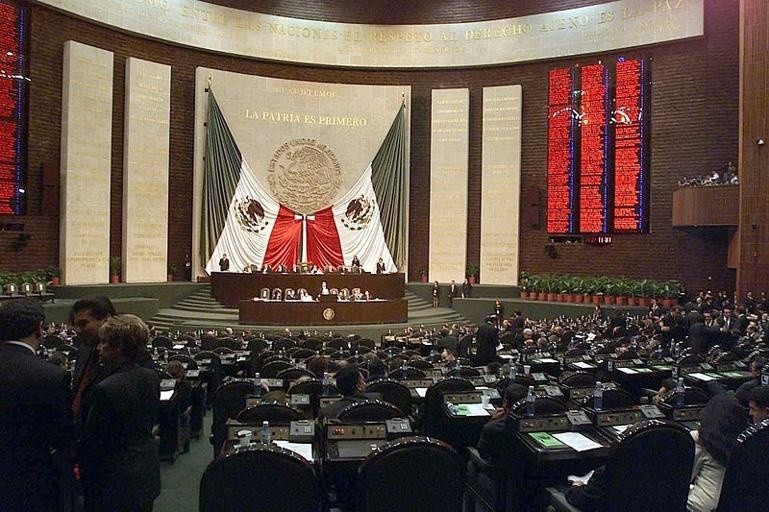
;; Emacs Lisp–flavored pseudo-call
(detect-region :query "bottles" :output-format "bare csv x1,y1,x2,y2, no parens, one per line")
154,315,685,445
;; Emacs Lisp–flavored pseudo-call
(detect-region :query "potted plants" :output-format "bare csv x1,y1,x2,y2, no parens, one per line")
519,270,683,306
111,256,121,284
467,263,479,284
418,266,428,283
0,265,60,295
167,264,176,281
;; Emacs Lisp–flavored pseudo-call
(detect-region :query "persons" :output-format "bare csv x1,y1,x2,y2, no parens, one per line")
352,256,364,274
678,160,738,187
219,253,229,271
272,280,362,300
0,290,769,512
377,257,387,275
245,261,347,273
432,277,473,309
